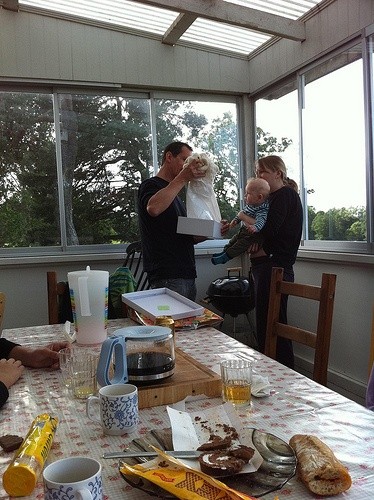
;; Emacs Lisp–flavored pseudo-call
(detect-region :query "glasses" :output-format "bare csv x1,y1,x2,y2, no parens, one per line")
256,169,274,174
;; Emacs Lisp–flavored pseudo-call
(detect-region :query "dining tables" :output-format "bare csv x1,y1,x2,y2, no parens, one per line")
0,318,374,500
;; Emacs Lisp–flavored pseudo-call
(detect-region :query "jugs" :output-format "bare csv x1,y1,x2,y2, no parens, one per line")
97,324,175,387
68,271,109,344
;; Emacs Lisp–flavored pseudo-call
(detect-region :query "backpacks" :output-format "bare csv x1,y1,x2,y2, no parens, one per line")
107,267,137,321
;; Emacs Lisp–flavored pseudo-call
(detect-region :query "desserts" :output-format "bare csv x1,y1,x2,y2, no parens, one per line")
195,436,254,476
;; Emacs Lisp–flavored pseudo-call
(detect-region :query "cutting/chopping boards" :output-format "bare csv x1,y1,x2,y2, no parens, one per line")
95,346,220,409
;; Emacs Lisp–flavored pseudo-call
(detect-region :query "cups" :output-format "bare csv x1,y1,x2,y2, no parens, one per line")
42,458,103,500
85,385,139,437
59,348,87,388
220,360,252,404
70,354,98,399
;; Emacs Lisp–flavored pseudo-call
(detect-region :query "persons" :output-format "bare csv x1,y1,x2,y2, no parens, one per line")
0,337,72,406
248,155,303,370
137,142,230,302
211,178,272,264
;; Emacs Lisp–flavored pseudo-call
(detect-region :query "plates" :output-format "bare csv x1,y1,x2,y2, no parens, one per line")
118,428,297,500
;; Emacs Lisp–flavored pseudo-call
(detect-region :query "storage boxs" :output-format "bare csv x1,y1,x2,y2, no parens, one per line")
176,216,225,238
121,287,205,322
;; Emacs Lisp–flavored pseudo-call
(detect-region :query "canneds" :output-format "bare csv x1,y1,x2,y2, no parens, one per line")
154,315,175,343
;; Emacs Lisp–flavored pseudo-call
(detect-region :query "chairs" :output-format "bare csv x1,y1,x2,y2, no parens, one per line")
265,268,336,386
47,241,152,324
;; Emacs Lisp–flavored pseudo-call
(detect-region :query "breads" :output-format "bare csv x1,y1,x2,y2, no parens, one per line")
0,434,23,452
289,434,352,496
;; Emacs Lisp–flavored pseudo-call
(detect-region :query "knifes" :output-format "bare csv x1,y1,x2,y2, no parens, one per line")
103,452,205,458
